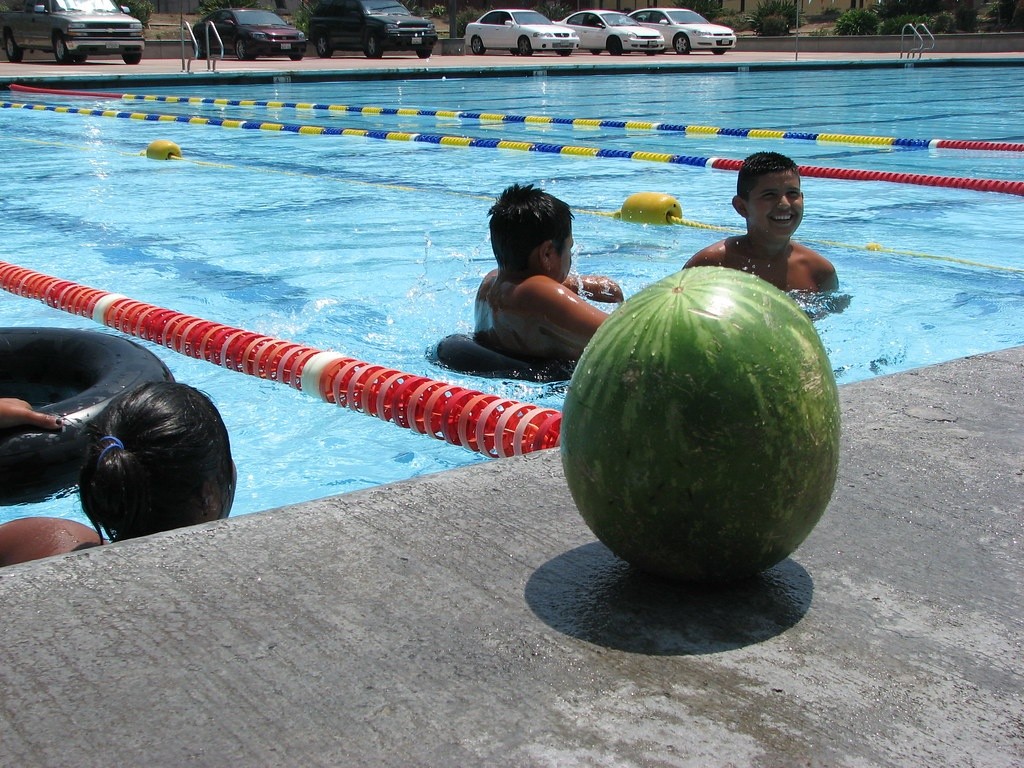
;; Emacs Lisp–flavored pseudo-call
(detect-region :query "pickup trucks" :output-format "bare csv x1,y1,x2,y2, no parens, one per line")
0,0,144,66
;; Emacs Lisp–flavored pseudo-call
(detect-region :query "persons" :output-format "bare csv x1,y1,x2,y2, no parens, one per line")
0,379,238,570
474,184,625,364
682,151,839,299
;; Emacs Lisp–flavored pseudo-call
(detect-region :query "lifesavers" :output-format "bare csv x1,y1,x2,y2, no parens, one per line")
433,329,567,383
0,326,175,505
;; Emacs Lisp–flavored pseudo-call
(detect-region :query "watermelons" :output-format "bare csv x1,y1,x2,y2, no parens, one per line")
560,266,840,585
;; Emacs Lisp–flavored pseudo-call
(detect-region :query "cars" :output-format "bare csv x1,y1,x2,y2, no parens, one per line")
610,8,737,56
190,7,308,62
464,8,580,56
552,10,666,56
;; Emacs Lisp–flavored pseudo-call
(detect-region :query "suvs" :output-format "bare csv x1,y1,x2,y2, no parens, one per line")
307,0,439,60
